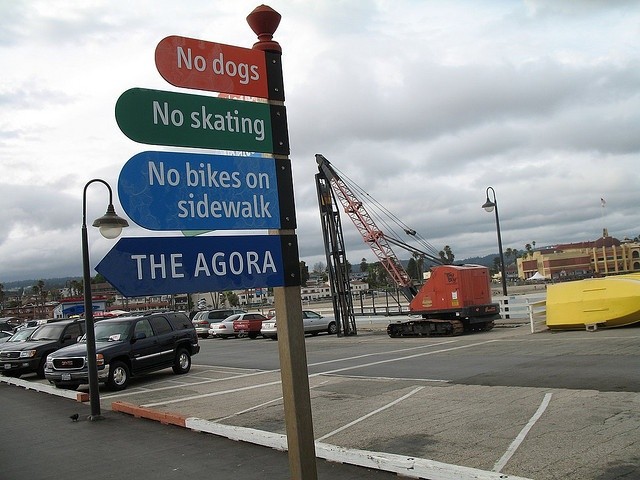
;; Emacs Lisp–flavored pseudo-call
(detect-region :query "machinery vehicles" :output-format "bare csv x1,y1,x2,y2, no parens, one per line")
315,153,502,339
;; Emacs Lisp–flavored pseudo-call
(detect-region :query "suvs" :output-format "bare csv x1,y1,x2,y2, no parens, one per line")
0,316,109,377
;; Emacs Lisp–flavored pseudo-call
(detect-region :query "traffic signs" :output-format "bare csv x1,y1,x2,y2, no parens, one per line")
115,87,290,157
154,35,285,101
94,234,301,298
118,151,297,232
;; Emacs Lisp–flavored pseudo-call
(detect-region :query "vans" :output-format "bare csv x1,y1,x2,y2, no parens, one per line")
44,309,202,389
192,309,246,338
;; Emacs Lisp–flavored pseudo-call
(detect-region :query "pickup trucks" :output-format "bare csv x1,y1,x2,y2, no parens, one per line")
233,319,267,339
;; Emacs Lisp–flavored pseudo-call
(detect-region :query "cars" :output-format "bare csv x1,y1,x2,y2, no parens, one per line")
208,312,266,339
0,327,38,344
260,310,336,341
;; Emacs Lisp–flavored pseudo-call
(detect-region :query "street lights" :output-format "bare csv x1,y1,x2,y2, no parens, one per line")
482,187,510,319
81,179,130,420
